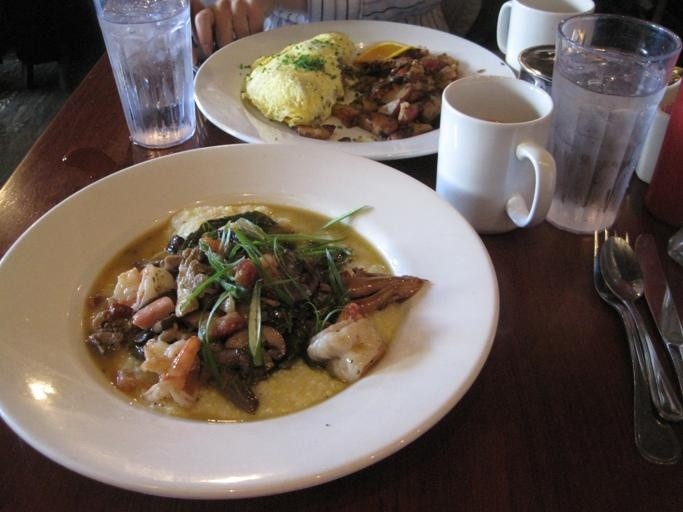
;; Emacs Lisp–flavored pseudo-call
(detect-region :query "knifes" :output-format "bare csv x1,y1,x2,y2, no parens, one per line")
632,232,682,392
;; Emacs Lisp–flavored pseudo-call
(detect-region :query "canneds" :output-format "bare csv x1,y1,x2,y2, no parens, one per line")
519,45,556,95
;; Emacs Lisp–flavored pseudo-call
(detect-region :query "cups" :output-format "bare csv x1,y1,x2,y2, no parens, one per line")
496,1,598,71
434,75,555,236
94,0,198,149
544,14,681,233
516,43,607,95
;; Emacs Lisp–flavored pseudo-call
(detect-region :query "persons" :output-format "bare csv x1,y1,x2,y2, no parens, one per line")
189,1,503,73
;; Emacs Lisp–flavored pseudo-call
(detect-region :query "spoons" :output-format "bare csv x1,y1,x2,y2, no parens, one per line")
598,234,682,422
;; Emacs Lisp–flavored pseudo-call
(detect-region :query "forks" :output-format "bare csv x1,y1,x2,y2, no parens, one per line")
585,224,683,467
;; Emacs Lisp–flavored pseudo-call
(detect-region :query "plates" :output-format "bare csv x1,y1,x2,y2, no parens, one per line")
0,143,502,499
191,20,519,162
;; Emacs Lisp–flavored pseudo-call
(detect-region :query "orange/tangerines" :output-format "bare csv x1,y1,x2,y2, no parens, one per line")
359,41,408,60
